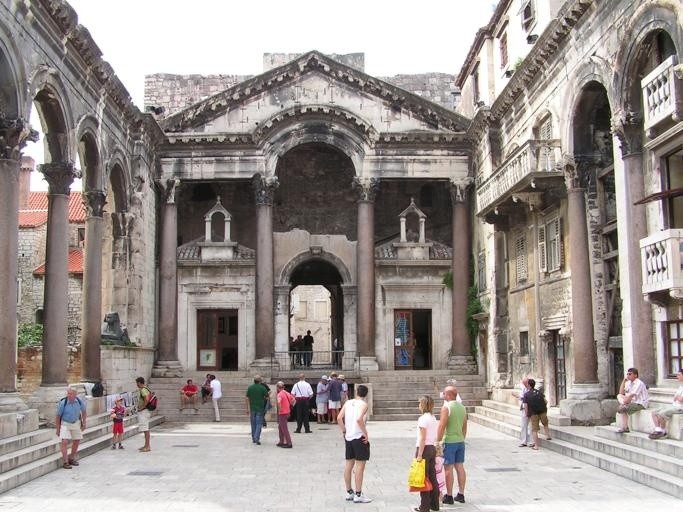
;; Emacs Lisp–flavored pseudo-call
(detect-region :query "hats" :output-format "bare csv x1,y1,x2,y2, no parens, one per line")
321,375,328,381
337,374,345,380
330,371,336,377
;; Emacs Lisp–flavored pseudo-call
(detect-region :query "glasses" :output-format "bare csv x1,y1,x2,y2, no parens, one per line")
627,373,632,375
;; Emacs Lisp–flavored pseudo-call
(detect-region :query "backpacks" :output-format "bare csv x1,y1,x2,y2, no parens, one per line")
139,387,157,412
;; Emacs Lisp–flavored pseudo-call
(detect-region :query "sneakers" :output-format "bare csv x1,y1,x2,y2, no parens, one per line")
528,443,535,447
212,419,219,422
113,445,117,449
650,430,663,435
527,445,539,450
442,495,454,504
202,400,207,404
345,490,356,501
281,445,294,448
255,438,261,445
141,448,150,452
455,493,465,503
179,406,186,410
63,463,72,469
615,426,631,434
139,446,145,450
193,407,197,410
353,493,372,503
430,504,438,511
275,443,282,446
317,420,323,425
322,421,328,424
546,434,552,442
69,459,79,466
519,443,527,447
648,431,668,441
414,507,430,512
118,445,123,449
294,429,301,433
305,430,313,433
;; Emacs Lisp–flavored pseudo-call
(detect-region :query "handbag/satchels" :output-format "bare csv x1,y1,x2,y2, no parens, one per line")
141,387,157,411
407,458,427,488
410,474,434,493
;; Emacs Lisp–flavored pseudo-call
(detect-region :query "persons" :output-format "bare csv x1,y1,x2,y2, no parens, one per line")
316,374,330,424
276,381,296,448
326,371,342,424
327,376,332,423
245,375,271,445
437,378,467,505
648,368,683,440
303,330,314,367
337,374,348,408
294,335,303,366
289,336,296,371
290,372,314,433
179,379,199,410
614,367,650,434
135,376,152,452
209,375,223,422
201,373,213,403
512,379,536,448
407,326,416,369
110,397,128,450
414,395,440,512
55,388,87,469
522,378,552,451
336,385,373,503
